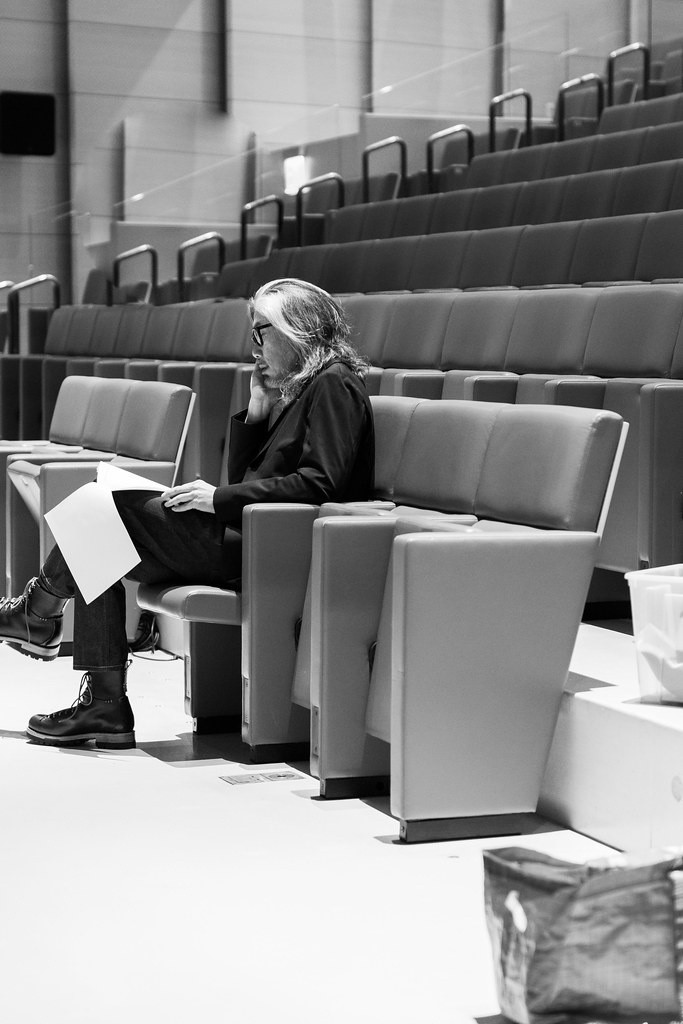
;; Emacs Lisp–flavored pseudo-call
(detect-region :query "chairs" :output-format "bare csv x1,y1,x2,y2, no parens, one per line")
2,41,682,842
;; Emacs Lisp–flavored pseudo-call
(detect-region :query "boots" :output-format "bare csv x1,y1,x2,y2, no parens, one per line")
26,659,135,751
0,578,74,661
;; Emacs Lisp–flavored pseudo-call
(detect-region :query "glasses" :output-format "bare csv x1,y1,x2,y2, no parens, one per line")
251,323,273,346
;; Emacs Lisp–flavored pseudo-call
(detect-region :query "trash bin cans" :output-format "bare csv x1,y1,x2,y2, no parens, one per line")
626,563,683,702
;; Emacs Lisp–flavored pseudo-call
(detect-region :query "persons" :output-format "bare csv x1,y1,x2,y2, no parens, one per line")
0,277,379,757
